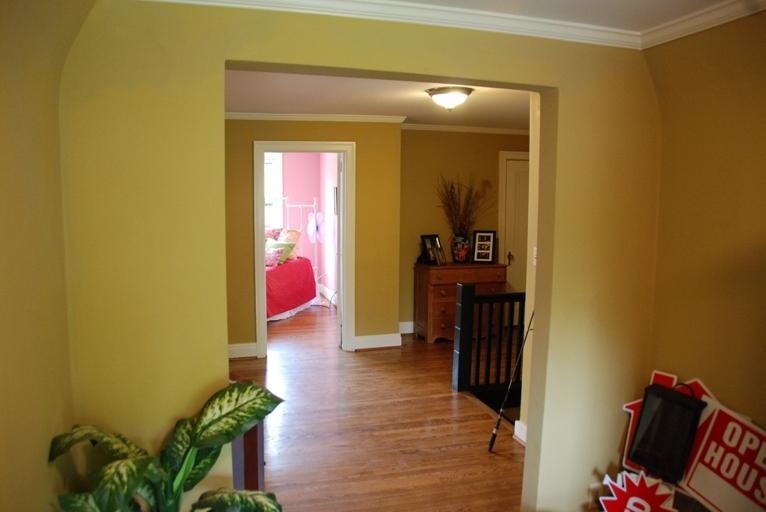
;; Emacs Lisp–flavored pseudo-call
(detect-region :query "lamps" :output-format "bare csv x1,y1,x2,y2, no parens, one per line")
426,86,474,113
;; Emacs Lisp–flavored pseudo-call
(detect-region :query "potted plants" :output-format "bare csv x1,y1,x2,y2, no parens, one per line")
450,233,470,263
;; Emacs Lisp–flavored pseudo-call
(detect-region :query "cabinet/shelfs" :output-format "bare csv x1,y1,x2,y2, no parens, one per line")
413,263,506,344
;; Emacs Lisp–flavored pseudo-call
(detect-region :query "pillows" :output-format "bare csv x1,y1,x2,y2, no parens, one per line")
266,228,301,266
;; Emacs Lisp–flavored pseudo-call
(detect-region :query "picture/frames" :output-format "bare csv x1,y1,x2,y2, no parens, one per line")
471,231,496,264
417,234,445,266
